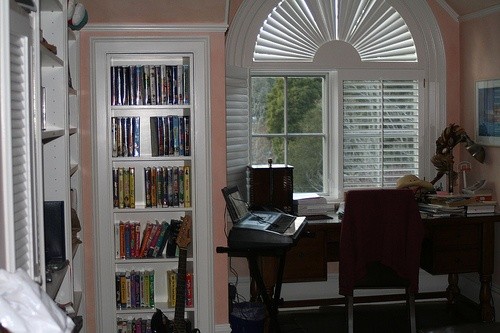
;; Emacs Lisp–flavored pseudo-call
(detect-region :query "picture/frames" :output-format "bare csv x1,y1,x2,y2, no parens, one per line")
474,77,500,147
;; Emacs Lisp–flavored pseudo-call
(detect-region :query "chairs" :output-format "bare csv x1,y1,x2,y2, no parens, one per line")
338,189,424,333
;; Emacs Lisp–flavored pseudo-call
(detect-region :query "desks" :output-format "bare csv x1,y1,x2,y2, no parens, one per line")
228,213,500,323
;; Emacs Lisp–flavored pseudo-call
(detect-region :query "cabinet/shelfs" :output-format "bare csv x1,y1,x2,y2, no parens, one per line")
34,1,86,333
106,52,197,332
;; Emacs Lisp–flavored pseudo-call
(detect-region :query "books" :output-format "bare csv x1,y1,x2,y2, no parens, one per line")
293,193,328,216
109,64,193,333
422,190,497,216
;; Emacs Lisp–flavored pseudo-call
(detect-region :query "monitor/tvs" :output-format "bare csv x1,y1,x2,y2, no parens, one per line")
44,200,65,264
